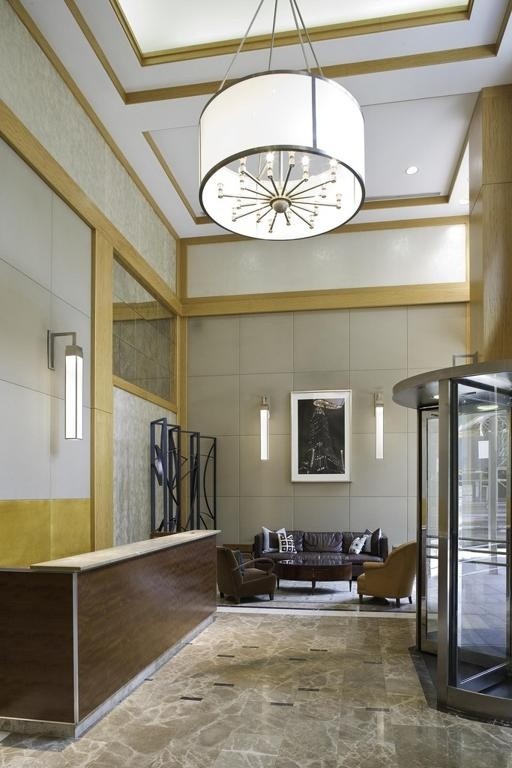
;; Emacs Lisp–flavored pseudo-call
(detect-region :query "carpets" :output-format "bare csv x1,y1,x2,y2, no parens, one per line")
216,579,416,612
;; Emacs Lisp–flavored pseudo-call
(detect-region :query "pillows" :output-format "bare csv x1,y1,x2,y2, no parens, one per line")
346,528,382,555
261,525,297,555
231,549,244,571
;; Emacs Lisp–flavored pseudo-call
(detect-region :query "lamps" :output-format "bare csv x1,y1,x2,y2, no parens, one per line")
259,395,270,462
193,0,364,242
46,329,84,440
373,391,385,460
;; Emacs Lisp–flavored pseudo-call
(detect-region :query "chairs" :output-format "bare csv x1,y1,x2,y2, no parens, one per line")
356,540,416,608
216,546,277,605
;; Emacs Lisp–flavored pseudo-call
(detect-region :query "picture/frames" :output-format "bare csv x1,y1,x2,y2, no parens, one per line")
291,390,351,483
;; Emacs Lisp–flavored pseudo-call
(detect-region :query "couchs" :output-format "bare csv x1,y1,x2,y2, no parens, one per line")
251,531,389,581
